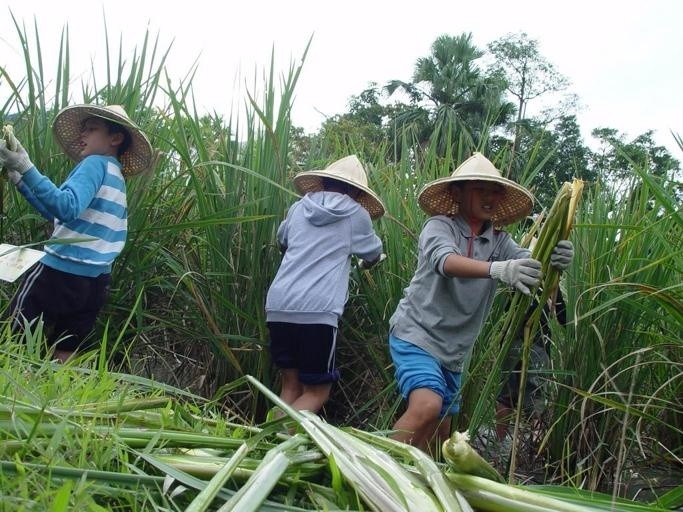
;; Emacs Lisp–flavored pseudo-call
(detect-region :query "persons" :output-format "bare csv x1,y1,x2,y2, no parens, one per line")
264,153,387,438
383,148,575,463
0,104,154,370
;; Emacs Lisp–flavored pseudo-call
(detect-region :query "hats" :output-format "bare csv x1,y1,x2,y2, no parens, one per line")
293,155,386,221
417,153,535,227
50,102,154,178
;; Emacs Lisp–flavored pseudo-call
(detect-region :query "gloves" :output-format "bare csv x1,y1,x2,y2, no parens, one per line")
488,258,543,297
549,239,575,273
0,126,34,188
